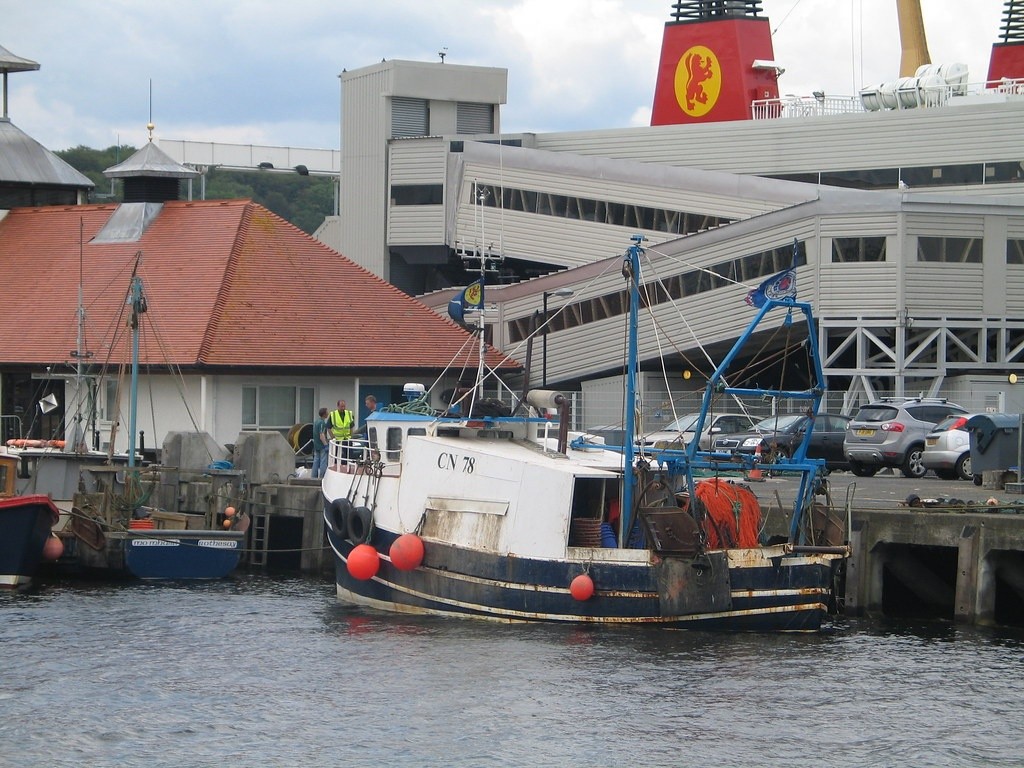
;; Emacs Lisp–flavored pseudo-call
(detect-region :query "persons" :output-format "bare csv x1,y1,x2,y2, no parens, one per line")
311,395,387,479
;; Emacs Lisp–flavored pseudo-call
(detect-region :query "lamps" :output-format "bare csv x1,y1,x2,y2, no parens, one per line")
814,91,824,97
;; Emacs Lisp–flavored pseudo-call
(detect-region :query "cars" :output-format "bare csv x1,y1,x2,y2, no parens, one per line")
922,409,1023,483
716,412,855,477
635,410,767,471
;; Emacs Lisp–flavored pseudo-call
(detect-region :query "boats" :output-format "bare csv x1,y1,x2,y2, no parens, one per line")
0,491,60,589
17,252,250,583
319,95,852,634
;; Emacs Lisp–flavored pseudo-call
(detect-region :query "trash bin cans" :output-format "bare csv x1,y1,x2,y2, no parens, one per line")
963,412,1020,486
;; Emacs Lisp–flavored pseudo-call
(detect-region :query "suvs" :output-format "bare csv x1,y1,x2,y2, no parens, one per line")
841,395,971,478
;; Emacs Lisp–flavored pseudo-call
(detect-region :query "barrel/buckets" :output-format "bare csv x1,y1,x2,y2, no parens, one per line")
572,518,645,548
129,520,154,529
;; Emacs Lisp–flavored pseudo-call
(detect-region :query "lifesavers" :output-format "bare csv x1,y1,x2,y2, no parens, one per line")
591,492,619,528
329,494,352,540
346,507,376,545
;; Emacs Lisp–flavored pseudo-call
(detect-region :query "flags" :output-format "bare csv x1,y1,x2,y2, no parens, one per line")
744,266,798,310
447,278,484,330
